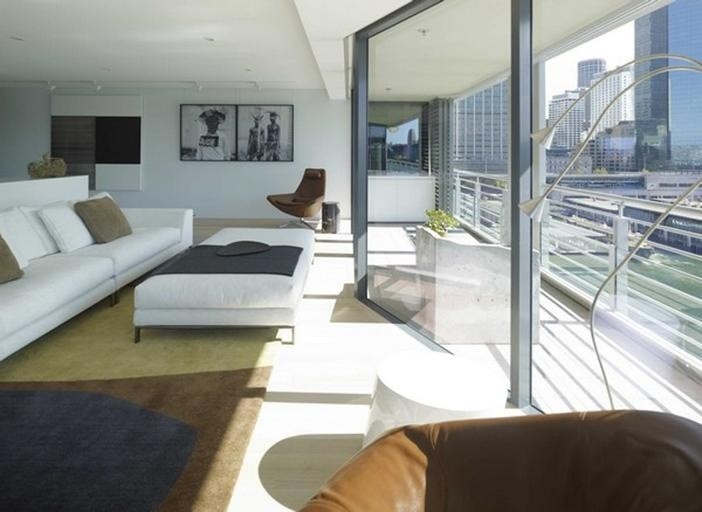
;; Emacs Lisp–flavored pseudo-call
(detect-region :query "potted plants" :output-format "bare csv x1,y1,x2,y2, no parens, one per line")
424,206,457,236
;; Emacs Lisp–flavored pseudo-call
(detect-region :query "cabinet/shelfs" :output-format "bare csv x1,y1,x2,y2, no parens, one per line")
368,177,435,223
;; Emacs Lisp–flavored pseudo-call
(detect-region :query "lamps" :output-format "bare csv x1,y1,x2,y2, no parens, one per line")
515,48,702,410
48,83,103,94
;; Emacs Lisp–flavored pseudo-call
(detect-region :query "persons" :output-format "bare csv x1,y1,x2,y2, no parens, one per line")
248,113,266,161
266,111,281,160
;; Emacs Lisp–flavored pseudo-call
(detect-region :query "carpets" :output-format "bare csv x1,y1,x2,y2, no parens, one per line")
1,217,315,511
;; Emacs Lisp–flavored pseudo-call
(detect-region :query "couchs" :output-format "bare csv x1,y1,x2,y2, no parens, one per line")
1,205,196,366
132,225,316,346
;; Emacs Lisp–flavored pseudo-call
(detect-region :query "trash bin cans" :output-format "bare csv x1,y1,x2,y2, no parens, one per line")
322,202,340,234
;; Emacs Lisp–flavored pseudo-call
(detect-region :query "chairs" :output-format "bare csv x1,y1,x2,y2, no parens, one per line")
265,168,326,233
293,410,702,510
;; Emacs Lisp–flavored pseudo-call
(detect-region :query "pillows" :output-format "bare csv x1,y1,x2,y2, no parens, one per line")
1,234,24,286
39,200,95,254
73,196,132,244
1,205,48,261
67,190,115,208
17,204,60,255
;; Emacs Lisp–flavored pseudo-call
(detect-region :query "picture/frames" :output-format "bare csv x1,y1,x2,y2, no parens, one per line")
180,103,294,162
